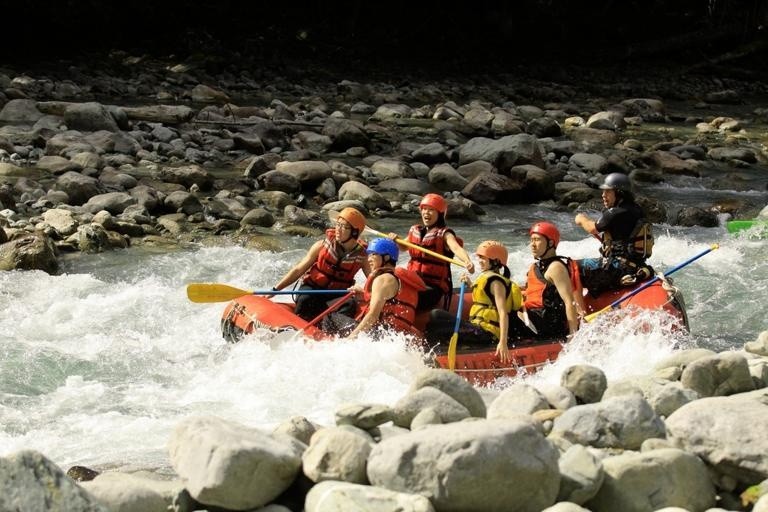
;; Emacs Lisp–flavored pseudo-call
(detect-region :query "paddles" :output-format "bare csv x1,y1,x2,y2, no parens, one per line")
329,211,465,266
446,284,466,370
269,290,354,350
584,243,719,322
518,294,538,335
187,284,350,304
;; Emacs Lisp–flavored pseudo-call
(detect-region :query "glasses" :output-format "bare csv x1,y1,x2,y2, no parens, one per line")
335,221,350,230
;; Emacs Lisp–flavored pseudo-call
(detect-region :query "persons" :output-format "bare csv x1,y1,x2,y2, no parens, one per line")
516,221,579,342
321,238,420,341
388,193,474,314
259,206,373,333
425,239,524,365
575,172,654,291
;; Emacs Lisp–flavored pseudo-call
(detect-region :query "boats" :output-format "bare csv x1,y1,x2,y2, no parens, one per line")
221,274,688,387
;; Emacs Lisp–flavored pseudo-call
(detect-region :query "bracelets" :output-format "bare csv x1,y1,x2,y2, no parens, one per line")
270,287,279,294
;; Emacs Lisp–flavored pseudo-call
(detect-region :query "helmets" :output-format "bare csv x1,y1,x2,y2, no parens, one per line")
336,207,366,236
418,192,448,214
474,240,507,266
529,222,560,248
364,237,399,261
599,173,632,199
